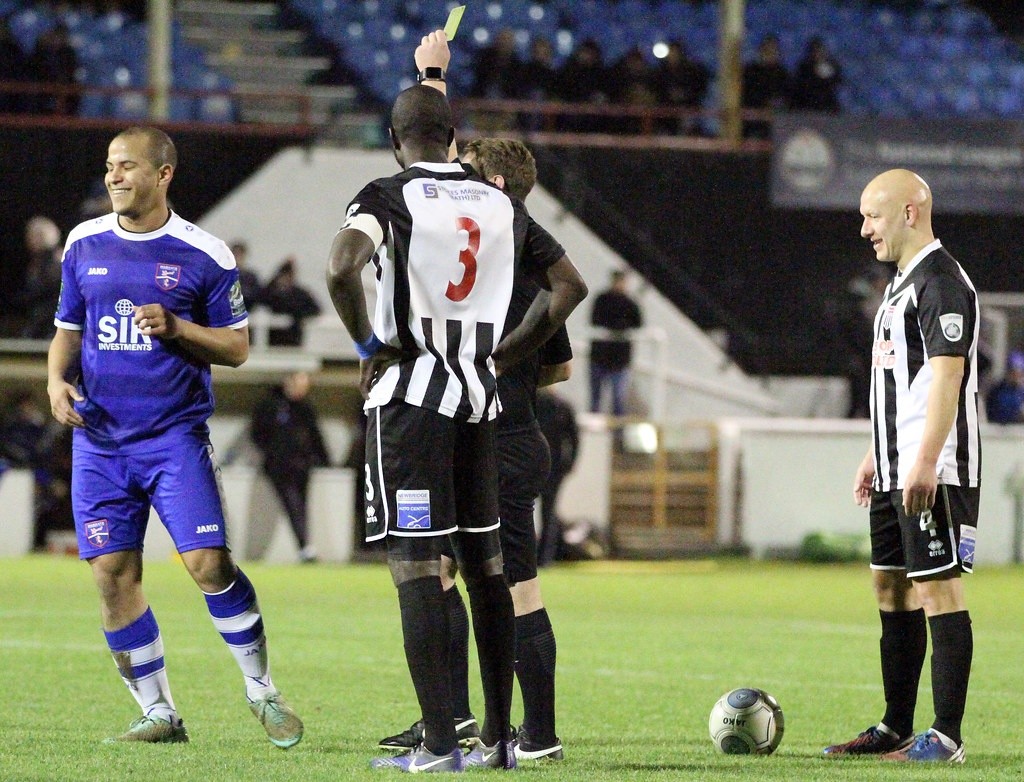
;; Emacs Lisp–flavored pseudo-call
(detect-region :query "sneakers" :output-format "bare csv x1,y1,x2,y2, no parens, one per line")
378,711,480,750
880,732,966,766
462,737,517,770
105,715,190,746
508,726,564,764
245,687,304,747
371,738,464,774
821,725,915,759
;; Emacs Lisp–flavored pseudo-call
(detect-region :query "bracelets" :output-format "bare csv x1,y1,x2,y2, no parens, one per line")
354,331,382,359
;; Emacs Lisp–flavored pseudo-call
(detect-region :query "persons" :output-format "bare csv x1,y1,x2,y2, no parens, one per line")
47,124,303,749
826,169,981,766
325,84,590,774
379,28,572,760
0,0,1024,567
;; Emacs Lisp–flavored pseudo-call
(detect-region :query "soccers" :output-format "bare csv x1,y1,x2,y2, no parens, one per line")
708,690,787,755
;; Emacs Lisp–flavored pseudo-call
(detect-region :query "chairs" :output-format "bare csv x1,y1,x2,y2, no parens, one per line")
0,1,1023,132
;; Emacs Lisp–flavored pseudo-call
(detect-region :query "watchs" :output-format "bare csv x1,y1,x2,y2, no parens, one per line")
418,67,447,83
